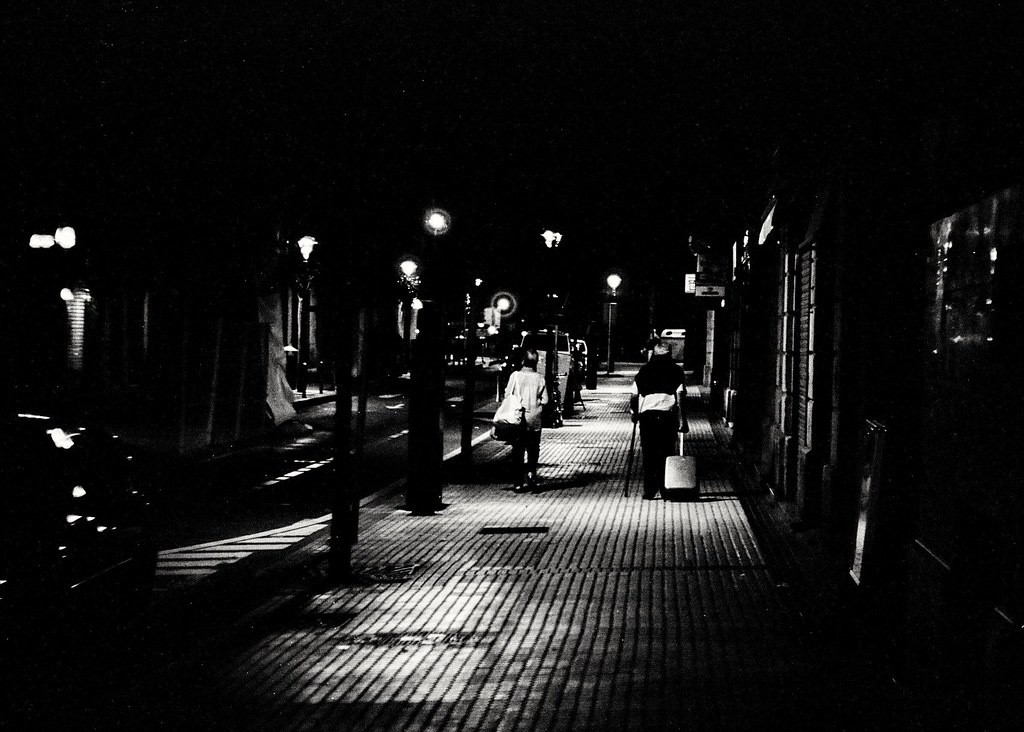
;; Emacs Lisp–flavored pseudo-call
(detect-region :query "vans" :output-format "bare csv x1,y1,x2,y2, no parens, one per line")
512,329,598,419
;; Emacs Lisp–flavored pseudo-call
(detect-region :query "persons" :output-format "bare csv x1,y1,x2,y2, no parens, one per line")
630,342,690,500
504,349,548,492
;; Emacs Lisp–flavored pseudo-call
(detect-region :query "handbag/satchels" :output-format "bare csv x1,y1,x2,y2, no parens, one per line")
493,395,528,443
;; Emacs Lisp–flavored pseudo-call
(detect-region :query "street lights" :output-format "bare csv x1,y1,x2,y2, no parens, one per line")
606,272,622,377
398,259,420,372
298,235,318,400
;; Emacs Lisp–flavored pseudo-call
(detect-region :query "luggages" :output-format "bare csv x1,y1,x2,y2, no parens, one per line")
663,431,700,501
